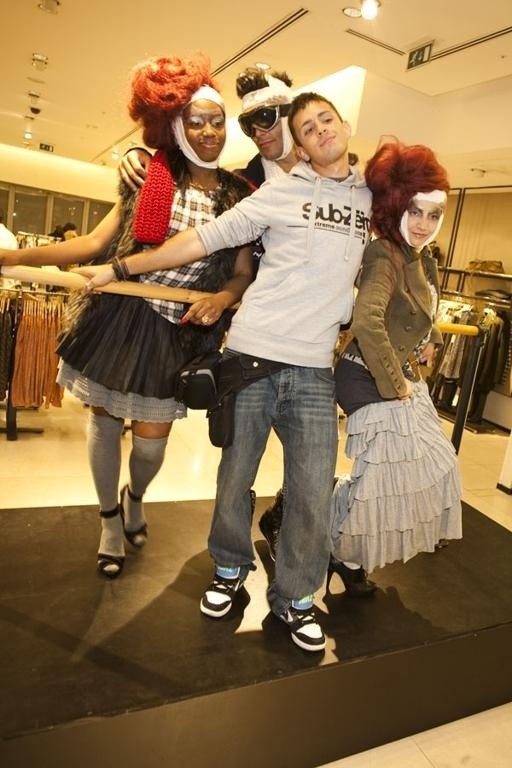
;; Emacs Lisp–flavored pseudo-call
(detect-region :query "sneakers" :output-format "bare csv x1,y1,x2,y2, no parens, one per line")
200,564,253,619
273,593,327,652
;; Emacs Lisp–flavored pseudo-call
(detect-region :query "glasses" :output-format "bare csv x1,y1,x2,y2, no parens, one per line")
241,106,290,138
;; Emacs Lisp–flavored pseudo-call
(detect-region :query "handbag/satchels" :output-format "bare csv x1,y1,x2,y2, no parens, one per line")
171,355,242,446
466,259,504,273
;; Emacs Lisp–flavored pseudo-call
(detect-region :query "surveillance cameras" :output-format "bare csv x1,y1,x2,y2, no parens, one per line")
30,107,40,115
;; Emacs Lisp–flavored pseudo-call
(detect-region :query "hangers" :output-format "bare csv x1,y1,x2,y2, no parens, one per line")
0,289,70,321
441,293,498,319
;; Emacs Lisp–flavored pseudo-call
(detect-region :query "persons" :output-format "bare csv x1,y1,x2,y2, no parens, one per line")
0,52,256,580
75,89,373,659
322,136,465,600
115,67,303,564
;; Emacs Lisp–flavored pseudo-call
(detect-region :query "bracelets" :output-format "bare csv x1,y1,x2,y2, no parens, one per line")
113,255,130,283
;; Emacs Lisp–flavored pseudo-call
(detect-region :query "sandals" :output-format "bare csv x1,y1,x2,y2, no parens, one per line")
98,505,124,579
119,485,148,549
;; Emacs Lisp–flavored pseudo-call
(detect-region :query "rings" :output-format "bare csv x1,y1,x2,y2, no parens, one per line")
202,315,210,325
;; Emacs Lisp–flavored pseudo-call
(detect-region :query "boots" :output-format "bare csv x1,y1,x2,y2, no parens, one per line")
258,489,283,564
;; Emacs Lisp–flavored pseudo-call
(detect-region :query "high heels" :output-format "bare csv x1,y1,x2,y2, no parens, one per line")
326,553,380,598
